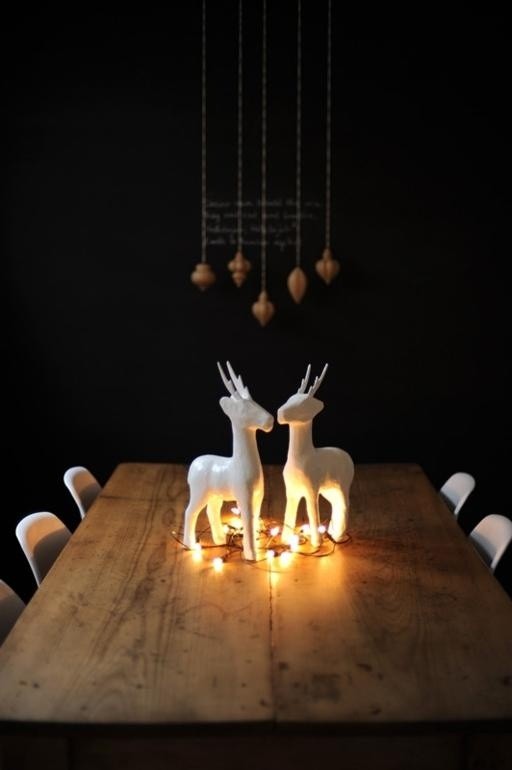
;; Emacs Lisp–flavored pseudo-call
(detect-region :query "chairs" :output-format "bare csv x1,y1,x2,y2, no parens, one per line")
438,471,512,575
1,466,102,645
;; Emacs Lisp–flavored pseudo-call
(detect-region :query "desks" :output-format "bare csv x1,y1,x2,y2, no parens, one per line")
1,462,512,770
